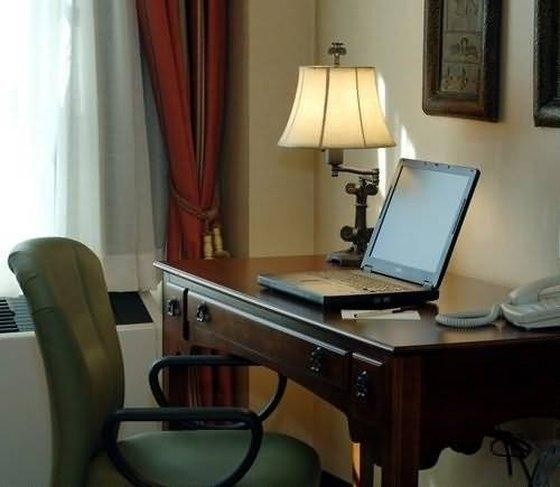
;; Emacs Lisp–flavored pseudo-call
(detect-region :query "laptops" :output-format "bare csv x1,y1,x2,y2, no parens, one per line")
256,158,481,308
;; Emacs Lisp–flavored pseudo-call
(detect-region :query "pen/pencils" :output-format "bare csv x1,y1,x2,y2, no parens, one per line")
353,306,406,318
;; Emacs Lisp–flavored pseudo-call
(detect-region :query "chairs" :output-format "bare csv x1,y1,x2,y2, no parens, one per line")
8,237,322,487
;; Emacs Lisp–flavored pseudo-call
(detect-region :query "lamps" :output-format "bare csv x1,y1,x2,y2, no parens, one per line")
277,42,396,269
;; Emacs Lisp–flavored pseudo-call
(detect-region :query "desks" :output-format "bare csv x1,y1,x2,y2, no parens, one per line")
153,255,560,487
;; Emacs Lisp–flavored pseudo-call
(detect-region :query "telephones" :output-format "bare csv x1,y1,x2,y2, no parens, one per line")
501,274,560,331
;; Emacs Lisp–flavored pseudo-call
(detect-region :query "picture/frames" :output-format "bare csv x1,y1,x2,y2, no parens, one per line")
531,0,560,128
422,0,502,122
